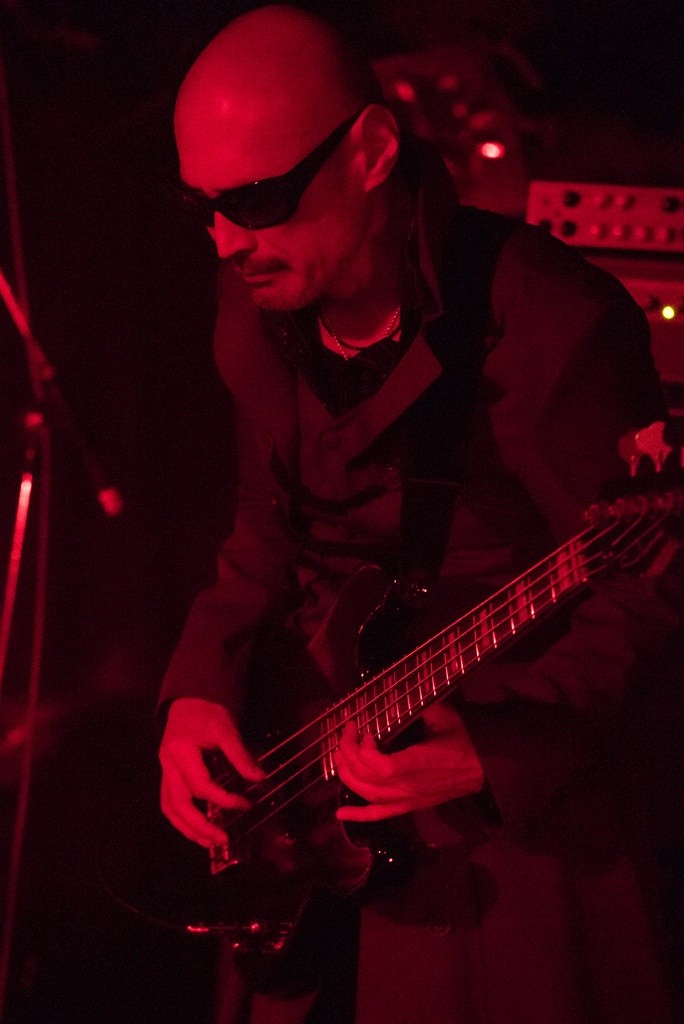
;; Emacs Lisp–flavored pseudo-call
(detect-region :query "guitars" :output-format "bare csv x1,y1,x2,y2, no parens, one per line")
194,414,684,994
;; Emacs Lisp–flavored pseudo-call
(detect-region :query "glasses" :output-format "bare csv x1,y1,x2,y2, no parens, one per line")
176,112,361,230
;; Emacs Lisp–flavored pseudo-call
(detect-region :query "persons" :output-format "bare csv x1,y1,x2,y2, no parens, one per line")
159,8,684,1024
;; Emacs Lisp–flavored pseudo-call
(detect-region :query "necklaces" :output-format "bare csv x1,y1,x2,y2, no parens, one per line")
323,303,400,362
318,308,400,352
